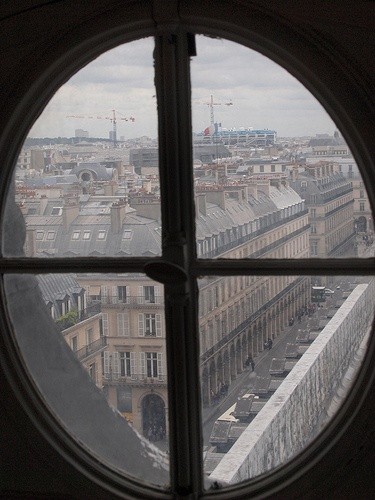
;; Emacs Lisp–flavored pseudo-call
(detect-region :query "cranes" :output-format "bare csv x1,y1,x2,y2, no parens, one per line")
66,107,136,148
194,94,234,134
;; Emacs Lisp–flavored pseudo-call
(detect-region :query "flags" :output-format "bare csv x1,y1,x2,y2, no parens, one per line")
204,123,218,136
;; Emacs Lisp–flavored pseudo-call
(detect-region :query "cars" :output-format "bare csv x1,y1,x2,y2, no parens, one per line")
320,289,335,294
